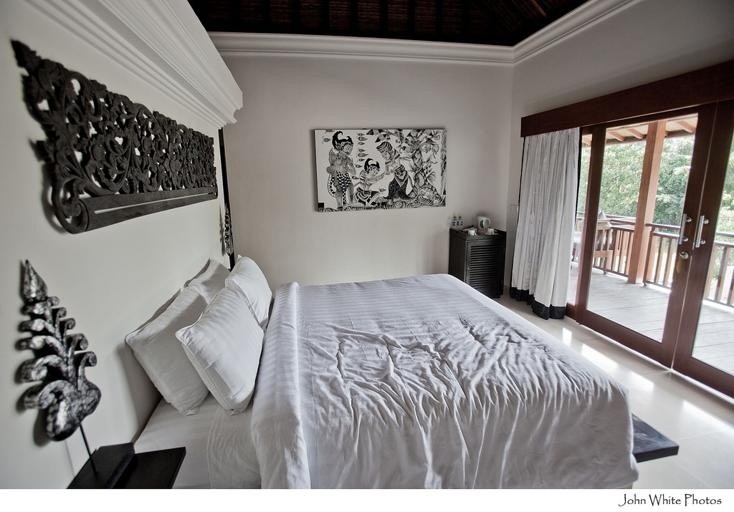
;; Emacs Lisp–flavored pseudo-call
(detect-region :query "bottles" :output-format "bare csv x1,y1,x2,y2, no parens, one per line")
452,215,464,226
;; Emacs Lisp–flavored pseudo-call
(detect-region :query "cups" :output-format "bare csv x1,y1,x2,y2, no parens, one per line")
467,229,475,236
487,228,493,235
478,216,490,231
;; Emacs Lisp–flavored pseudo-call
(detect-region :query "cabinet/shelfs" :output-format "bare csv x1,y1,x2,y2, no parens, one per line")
445,227,507,299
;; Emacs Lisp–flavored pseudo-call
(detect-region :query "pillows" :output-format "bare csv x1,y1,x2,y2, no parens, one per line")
172,286,264,418
186,258,229,306
224,253,275,329
125,286,212,418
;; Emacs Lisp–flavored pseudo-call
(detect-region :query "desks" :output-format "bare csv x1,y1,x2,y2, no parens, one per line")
66,440,186,487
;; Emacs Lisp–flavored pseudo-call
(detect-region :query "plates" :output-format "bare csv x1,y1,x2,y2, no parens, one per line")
485,233,498,236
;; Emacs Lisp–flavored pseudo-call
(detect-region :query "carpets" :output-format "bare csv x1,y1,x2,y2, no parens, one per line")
629,412,678,465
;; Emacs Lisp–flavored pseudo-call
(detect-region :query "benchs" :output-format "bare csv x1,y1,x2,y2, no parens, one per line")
571,224,620,274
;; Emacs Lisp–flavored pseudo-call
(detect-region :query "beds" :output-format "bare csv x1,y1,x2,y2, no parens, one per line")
129,271,639,488
